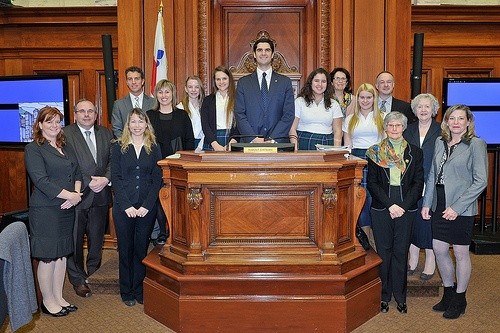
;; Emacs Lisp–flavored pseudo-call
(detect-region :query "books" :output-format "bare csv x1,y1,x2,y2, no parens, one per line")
244,147,278,153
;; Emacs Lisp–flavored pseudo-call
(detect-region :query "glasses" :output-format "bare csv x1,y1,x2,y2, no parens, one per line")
76,109,96,113
387,124,403,128
333,77,348,82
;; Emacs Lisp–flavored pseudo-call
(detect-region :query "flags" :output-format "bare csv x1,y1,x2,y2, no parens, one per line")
149,11,168,98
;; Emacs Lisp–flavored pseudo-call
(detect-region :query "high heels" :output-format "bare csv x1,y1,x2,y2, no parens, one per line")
63,304,77,311
41,302,68,316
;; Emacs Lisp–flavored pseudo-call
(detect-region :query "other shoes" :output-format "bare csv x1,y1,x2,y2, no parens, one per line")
397,301,407,313
407,261,419,275
156,233,166,245
123,298,136,306
381,301,387,313
421,272,434,280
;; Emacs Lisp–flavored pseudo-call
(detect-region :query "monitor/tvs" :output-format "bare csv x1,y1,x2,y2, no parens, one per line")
0,74,69,149
441,78,500,151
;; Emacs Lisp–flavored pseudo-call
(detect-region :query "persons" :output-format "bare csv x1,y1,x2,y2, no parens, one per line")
421,105,488,320
60,98,114,297
289,67,343,152
375,71,418,139
234,38,295,144
24,105,84,317
175,75,206,149
365,111,425,314
329,68,356,147
402,93,443,280
201,66,241,151
112,67,165,245
111,108,163,306
342,83,388,241
145,80,195,245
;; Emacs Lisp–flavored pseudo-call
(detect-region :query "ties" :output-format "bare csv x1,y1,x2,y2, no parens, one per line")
380,101,386,115
135,97,139,109
85,131,96,161
261,72,268,107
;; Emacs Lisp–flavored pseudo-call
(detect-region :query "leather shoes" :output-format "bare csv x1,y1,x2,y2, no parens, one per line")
74,284,90,297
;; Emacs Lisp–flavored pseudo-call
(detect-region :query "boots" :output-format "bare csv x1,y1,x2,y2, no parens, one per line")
432,283,456,311
443,290,467,318
